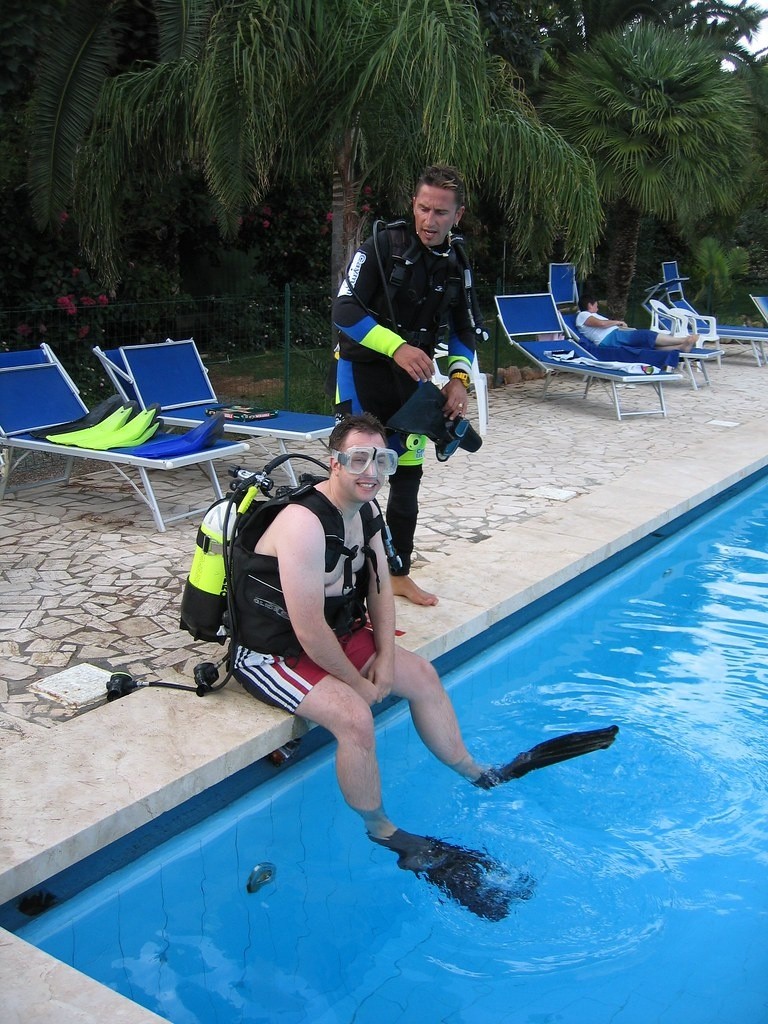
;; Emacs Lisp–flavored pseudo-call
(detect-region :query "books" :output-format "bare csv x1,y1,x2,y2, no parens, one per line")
205,405,279,422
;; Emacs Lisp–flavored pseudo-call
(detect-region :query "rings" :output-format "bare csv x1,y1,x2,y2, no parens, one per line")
458,403,463,408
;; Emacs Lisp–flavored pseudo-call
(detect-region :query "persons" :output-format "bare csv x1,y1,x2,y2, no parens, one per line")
329,161,477,607
228,412,468,812
575,294,700,353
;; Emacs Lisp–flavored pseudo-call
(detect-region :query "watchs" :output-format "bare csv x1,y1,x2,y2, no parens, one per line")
452,373,471,389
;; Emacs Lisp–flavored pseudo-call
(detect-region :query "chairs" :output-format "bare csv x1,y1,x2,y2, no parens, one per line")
0,337,335,533
470,261,768,437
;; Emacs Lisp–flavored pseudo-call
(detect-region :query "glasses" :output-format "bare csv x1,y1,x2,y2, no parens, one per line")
329,445,400,476
